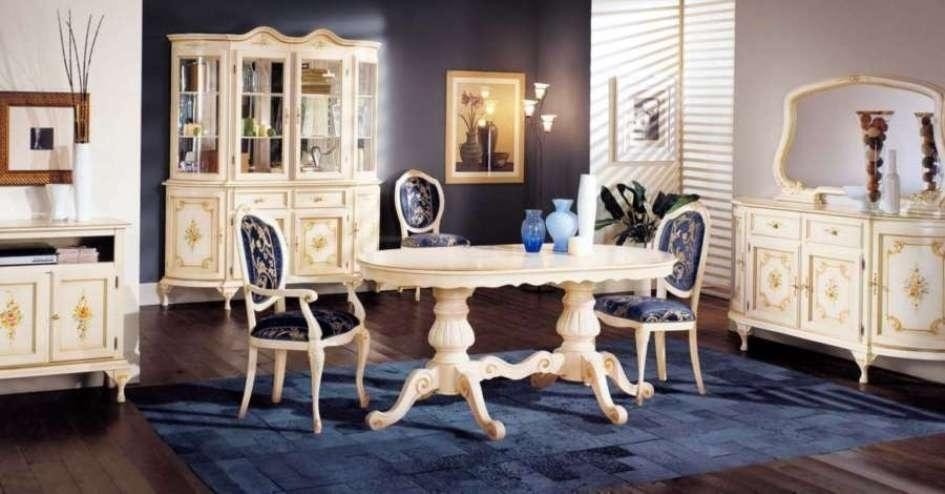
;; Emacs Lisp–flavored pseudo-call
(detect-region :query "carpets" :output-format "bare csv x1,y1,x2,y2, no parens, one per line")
124,338,945,494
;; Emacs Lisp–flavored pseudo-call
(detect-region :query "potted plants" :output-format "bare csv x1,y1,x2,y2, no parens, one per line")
591,179,699,295
53,7,105,224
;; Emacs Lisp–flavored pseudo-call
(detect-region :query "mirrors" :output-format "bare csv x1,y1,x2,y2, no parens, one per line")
773,74,945,208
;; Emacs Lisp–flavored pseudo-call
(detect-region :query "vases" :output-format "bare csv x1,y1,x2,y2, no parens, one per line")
521,207,546,254
544,199,581,251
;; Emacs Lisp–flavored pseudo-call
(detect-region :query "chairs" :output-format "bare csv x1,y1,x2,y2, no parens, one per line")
593,202,711,404
233,204,368,433
394,169,472,303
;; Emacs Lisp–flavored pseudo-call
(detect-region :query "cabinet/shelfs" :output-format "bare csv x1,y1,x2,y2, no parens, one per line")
0,222,132,403
158,27,381,311
726,197,944,386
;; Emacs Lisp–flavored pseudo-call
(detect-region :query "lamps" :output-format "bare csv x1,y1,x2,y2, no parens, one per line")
524,83,556,209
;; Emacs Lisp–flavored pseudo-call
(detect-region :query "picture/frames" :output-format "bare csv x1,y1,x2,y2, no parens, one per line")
446,70,525,185
0,91,90,186
611,77,672,165
